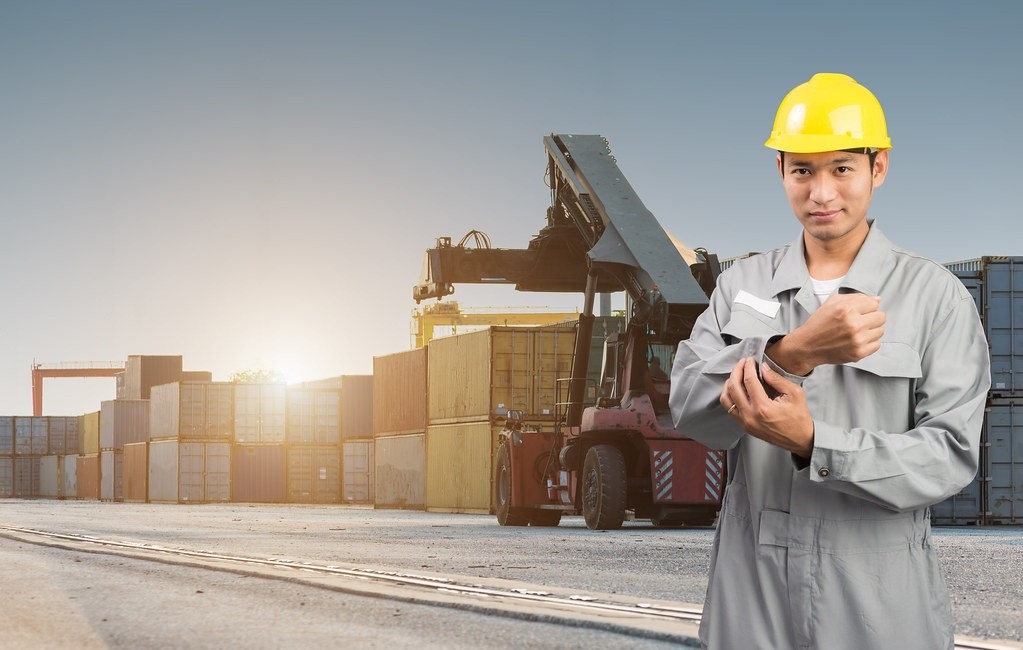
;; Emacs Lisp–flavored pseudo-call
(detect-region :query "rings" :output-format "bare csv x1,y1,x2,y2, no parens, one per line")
727,404,736,413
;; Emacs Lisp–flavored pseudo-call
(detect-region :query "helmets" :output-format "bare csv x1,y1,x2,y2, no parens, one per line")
764,72,893,155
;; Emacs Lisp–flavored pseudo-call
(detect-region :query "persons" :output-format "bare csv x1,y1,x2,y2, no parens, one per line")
670,72,991,650
649,357,667,383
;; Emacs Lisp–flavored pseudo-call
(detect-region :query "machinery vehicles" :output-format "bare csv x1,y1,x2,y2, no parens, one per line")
411,133,728,531
